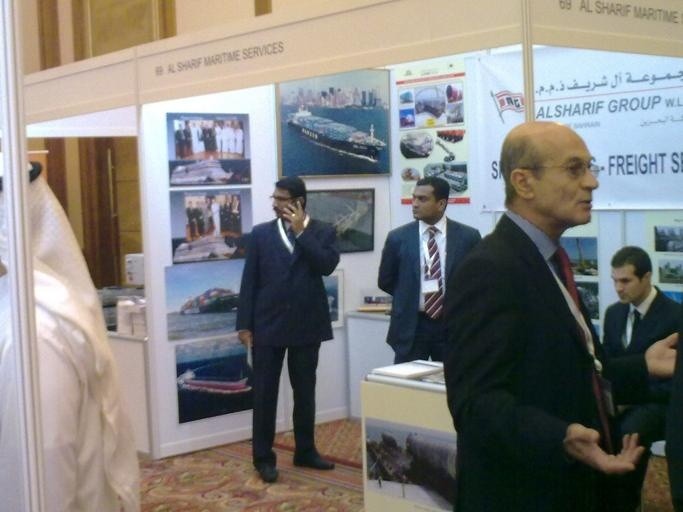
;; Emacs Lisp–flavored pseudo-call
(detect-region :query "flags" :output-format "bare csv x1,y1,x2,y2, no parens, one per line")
495,90,526,113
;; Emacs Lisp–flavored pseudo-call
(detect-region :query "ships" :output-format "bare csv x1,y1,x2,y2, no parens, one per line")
179,287,238,314
180,376,248,394
169,156,233,184
287,106,386,160
172,231,236,263
334,201,371,237
366,436,397,477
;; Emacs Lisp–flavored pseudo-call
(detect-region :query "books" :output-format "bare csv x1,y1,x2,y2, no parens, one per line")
372,358,444,379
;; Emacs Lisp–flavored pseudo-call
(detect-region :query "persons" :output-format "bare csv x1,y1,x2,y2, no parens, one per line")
176,119,246,158
375,175,482,366
601,244,681,511
0,149,143,510
235,174,341,486
186,194,241,239
441,117,679,511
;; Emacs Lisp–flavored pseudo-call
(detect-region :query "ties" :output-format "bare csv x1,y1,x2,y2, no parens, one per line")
554,246,614,454
424,226,444,319
630,309,641,343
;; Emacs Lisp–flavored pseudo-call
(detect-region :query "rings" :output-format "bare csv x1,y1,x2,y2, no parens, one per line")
291,213,295,217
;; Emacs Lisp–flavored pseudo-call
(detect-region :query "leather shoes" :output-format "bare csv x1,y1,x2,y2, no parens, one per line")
259,466,278,482
313,457,335,469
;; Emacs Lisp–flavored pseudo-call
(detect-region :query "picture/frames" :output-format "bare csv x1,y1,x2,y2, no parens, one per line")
275,69,392,181
322,269,345,331
304,188,376,253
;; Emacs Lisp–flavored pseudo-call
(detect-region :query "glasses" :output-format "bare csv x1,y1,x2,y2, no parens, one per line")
520,162,602,180
269,194,297,202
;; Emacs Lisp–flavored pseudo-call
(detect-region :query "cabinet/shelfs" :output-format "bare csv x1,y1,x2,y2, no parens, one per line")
361,374,459,512
